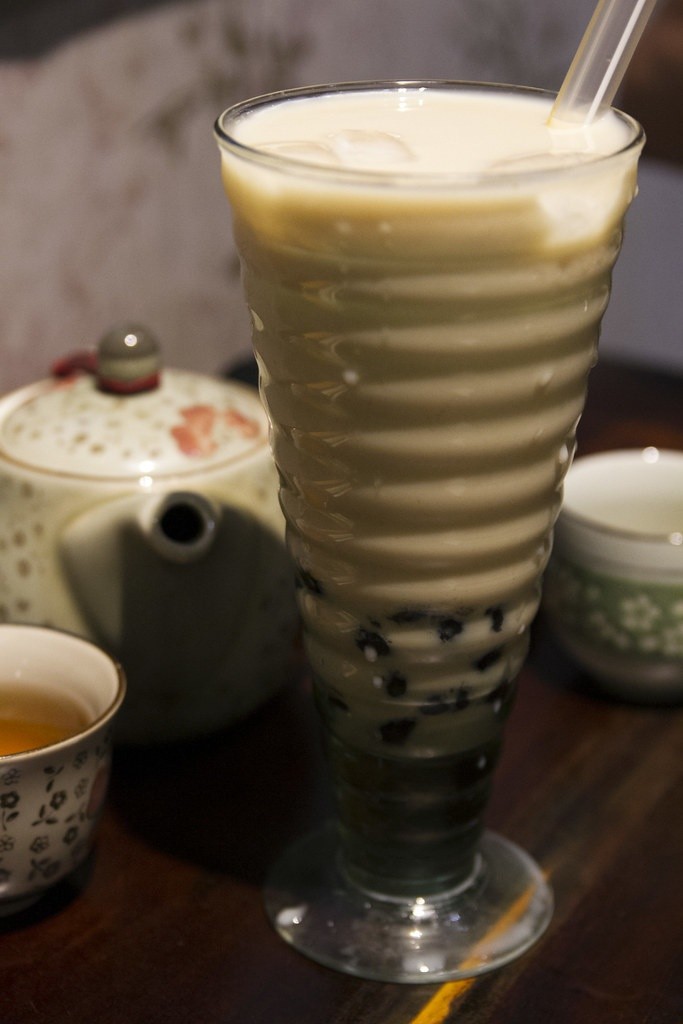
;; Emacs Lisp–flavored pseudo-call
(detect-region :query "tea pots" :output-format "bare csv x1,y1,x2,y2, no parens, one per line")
0,321,292,747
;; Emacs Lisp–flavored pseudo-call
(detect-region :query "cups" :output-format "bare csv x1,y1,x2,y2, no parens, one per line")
0,620,130,918
549,447,683,709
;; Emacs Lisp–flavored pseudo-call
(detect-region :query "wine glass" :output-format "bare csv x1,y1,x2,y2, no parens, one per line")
210,78,644,983
